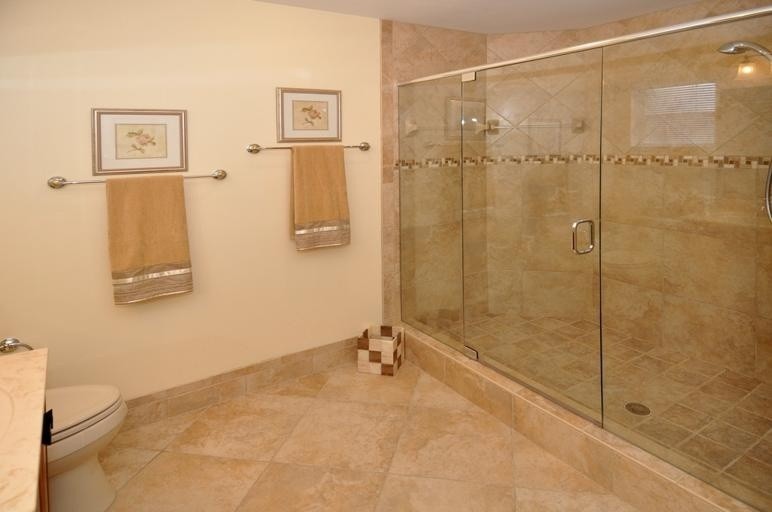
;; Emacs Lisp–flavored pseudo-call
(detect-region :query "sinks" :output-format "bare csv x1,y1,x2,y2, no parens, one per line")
0,387,14,438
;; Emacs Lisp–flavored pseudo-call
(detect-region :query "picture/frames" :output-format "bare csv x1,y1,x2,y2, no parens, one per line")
275,87,341,143
444,96,486,142
90,108,188,176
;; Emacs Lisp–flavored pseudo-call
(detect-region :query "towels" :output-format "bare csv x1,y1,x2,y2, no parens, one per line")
290,144,351,252
105,174,193,305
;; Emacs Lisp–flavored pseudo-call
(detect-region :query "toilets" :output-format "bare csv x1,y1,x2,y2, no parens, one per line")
46,385,128,512
